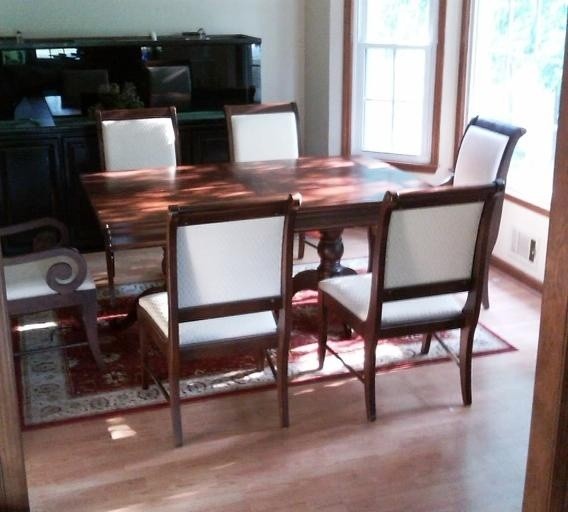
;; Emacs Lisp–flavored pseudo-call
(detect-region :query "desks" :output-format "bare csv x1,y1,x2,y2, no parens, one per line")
79,153,436,337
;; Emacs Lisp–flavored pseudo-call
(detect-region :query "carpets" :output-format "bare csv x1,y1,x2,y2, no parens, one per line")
10,257,518,430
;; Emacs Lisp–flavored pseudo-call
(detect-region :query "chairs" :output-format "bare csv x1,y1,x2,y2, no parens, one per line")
90,107,182,309
225,101,317,259
3,215,107,369
317,179,507,422
433,115,526,309
137,192,303,448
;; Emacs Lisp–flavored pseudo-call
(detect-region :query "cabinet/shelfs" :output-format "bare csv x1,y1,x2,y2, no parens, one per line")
0,132,100,238
